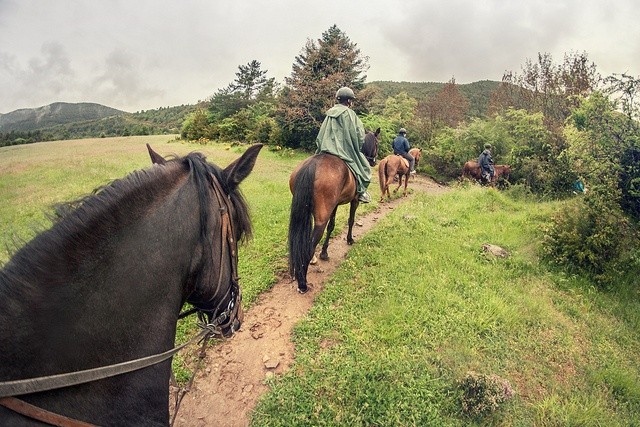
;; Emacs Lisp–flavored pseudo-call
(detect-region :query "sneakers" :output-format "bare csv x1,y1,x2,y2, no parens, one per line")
358,194,370,202
410,170,416,174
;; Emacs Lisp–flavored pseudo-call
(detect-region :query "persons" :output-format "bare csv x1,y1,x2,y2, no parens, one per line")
477,144,495,183
393,126,416,175
315,86,373,204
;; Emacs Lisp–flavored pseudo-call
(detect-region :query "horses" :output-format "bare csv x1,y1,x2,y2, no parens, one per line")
379,148,422,203
457,160,512,187
0,142,267,427
287,127,383,294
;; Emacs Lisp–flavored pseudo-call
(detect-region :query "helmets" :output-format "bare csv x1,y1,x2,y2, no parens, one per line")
484,144,491,148
399,128,407,133
335,87,356,100
